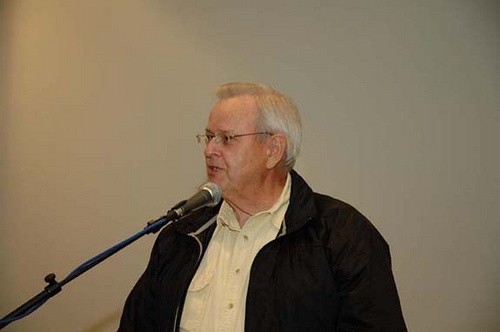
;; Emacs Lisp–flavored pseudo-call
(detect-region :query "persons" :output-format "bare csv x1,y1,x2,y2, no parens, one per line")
117,82,408,332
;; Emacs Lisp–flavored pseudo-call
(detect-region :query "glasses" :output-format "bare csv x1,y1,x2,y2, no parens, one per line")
196,131,274,147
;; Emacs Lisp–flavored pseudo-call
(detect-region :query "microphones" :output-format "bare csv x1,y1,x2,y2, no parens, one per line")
167,182,221,221
